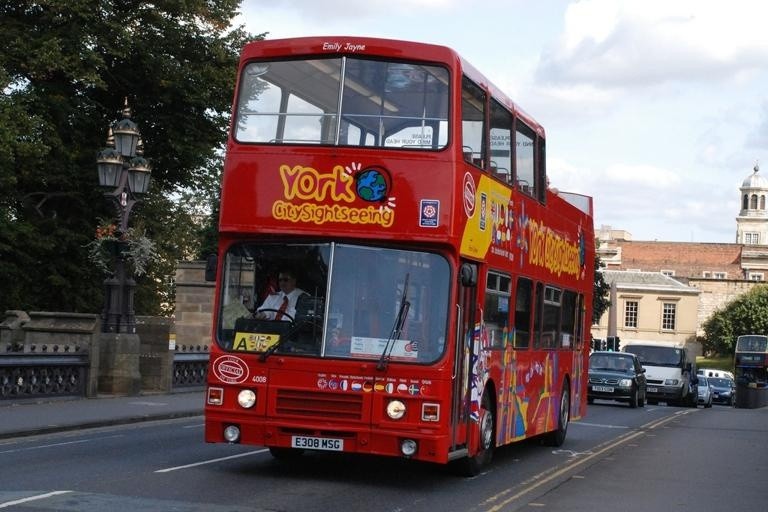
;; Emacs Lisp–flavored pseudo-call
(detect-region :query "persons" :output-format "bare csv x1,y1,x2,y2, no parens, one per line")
256,268,309,320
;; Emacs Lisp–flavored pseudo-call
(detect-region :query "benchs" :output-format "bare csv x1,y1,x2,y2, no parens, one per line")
408,143,533,194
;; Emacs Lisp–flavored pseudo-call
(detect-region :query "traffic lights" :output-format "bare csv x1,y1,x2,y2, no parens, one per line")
616,337,621,352
603,340,607,351
607,336,615,352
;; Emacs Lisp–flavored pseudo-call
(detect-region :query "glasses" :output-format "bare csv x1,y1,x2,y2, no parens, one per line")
279,278,291,282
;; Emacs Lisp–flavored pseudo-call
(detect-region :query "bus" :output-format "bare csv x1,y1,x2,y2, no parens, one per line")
201,37,595,477
734,334,768,389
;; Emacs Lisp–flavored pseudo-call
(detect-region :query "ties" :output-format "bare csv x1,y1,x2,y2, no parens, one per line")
275,298,288,320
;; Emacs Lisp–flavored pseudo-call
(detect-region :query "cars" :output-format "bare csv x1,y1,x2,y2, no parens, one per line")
588,340,736,410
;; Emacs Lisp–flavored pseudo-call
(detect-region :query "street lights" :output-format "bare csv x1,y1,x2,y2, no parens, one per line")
96,103,153,332
